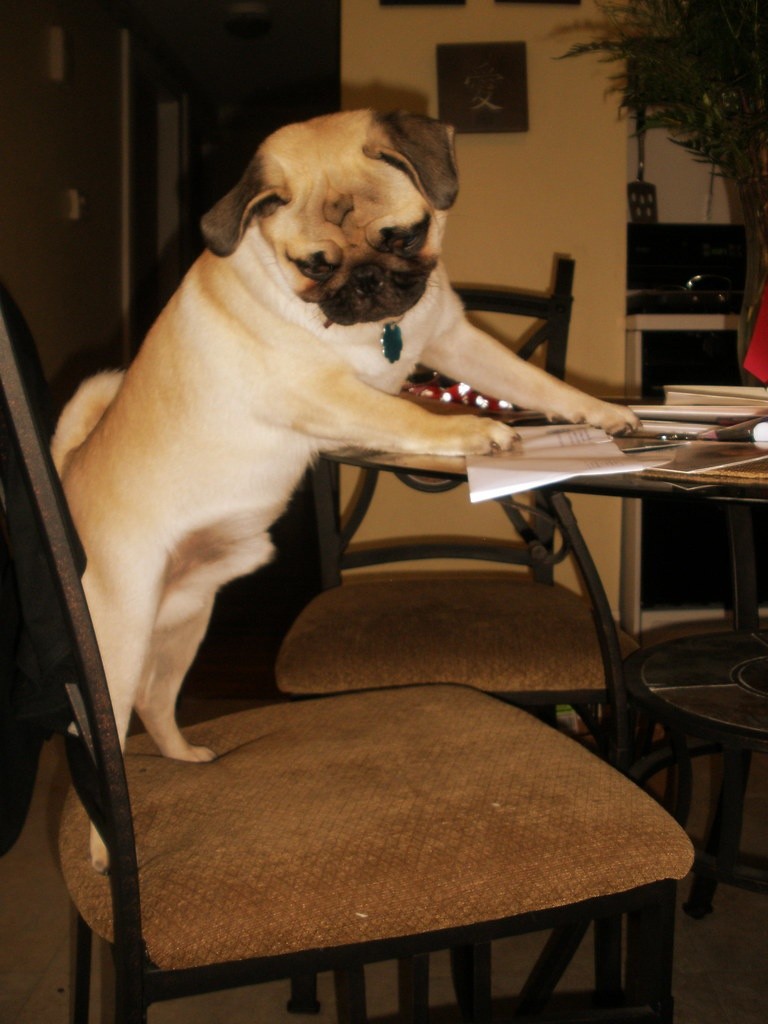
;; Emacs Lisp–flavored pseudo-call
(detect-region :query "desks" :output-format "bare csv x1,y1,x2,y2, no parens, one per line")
321,394,768,1024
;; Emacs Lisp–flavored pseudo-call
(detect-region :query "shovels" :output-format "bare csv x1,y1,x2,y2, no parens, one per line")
626,101,659,225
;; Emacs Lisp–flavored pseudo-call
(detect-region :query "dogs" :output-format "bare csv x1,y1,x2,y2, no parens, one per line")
48,98,645,876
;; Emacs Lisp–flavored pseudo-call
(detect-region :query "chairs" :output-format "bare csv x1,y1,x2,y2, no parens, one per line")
0,288,693,1024
275,257,642,1016
